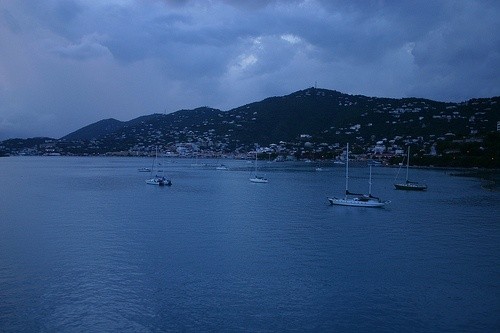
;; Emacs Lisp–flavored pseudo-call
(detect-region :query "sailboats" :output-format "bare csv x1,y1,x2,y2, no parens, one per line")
248,152,269,183
327,142,392,208
394,146,428,190
146,145,172,186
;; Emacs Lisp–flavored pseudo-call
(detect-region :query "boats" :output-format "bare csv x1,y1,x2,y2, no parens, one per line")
138,154,164,172
215,155,230,170
189,153,207,167
